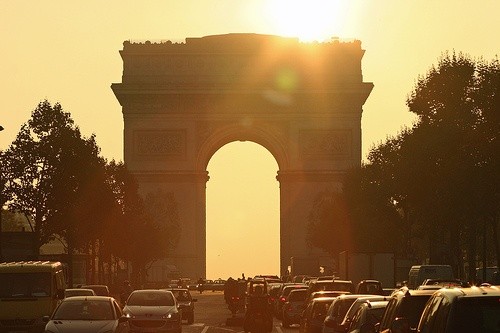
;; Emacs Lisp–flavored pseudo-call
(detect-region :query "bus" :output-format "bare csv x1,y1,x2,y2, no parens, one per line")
0,260,67,333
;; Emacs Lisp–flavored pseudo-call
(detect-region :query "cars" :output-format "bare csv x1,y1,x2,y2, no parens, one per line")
168,278,226,292
42,295,132,333
80,284,111,296
231,274,499,333
64,289,98,296
122,289,184,333
160,287,198,324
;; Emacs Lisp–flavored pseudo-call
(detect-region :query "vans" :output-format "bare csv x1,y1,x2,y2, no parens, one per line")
408,265,455,289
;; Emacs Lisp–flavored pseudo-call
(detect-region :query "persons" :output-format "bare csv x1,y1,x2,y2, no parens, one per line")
225,277,238,307
198,277,204,291
177,278,183,288
121,280,133,302
